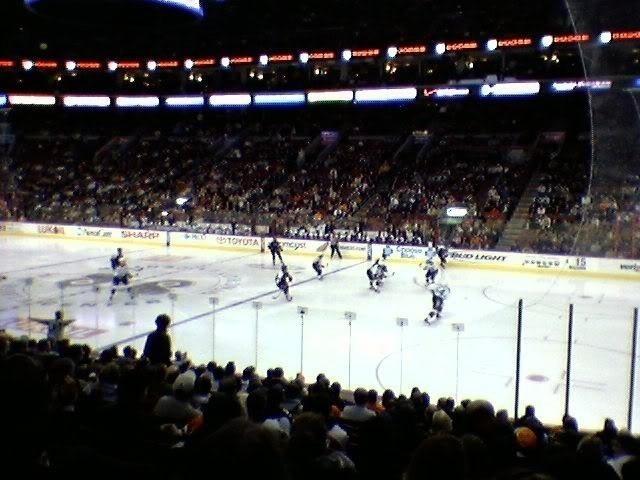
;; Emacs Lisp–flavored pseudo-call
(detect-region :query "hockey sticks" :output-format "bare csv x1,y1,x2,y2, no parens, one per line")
281,246,299,251
319,262,328,267
413,276,431,292
272,281,290,299
384,271,395,278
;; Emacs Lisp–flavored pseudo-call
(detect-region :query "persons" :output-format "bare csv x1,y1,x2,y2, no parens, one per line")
0,54,640,264
274,264,295,302
424,285,452,326
143,314,172,366
435,247,449,269
312,253,325,278
111,247,125,271
375,264,388,286
30,310,75,341
422,259,439,287
105,257,135,307
1,337,639,480
366,257,381,291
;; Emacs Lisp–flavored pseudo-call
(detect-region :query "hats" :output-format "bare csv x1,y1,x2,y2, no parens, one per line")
172,370,197,393
514,427,537,449
